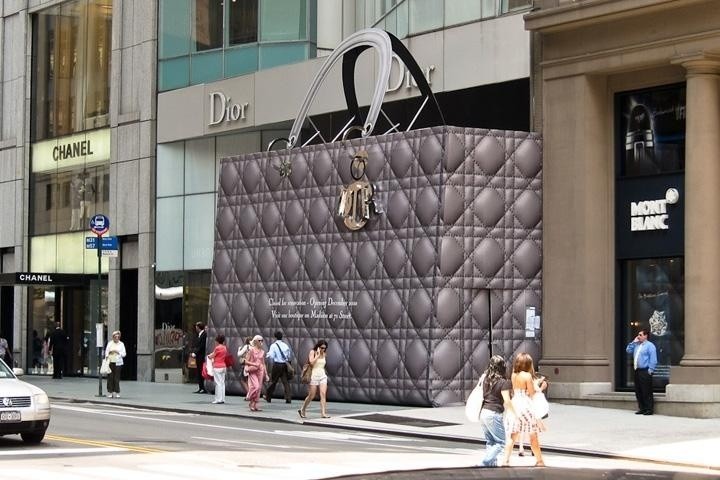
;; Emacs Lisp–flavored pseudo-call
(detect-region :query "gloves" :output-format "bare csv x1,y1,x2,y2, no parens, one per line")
191,352,196,358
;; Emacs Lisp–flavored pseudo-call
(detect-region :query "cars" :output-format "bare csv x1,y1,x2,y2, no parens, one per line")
0,355,53,448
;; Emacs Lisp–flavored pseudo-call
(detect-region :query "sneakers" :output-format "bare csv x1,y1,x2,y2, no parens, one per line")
211,400,225,404
107,393,122,399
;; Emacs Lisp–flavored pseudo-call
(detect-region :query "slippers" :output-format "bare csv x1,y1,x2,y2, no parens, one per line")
320,415,331,418
298,409,307,419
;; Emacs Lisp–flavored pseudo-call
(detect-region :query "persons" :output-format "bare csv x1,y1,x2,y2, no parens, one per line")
68,173,95,231
208,335,226,404
298,340,330,418
204,325,208,333
262,331,292,403
626,329,657,415
0,322,69,379
237,336,253,401
192,322,207,394
245,335,270,411
479,352,548,467
105,331,126,398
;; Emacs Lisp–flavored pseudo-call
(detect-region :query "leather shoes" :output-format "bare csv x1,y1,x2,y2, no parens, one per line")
634,410,645,414
192,389,201,393
643,409,654,415
285,399,291,404
199,389,205,394
263,394,271,403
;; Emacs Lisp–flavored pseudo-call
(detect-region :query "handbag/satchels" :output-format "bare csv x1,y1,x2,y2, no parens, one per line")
238,345,249,365
99,353,113,375
286,361,295,381
464,373,488,422
244,365,259,373
298,350,315,385
224,344,235,368
530,383,550,421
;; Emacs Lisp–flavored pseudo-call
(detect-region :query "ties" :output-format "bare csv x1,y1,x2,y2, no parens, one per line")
634,343,643,371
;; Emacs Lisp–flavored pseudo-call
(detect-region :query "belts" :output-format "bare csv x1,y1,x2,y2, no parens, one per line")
275,362,285,364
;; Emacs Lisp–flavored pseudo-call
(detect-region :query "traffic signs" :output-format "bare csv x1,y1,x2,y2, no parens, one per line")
84,235,118,250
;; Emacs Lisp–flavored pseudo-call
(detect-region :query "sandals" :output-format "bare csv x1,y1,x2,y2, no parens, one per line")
249,403,263,412
